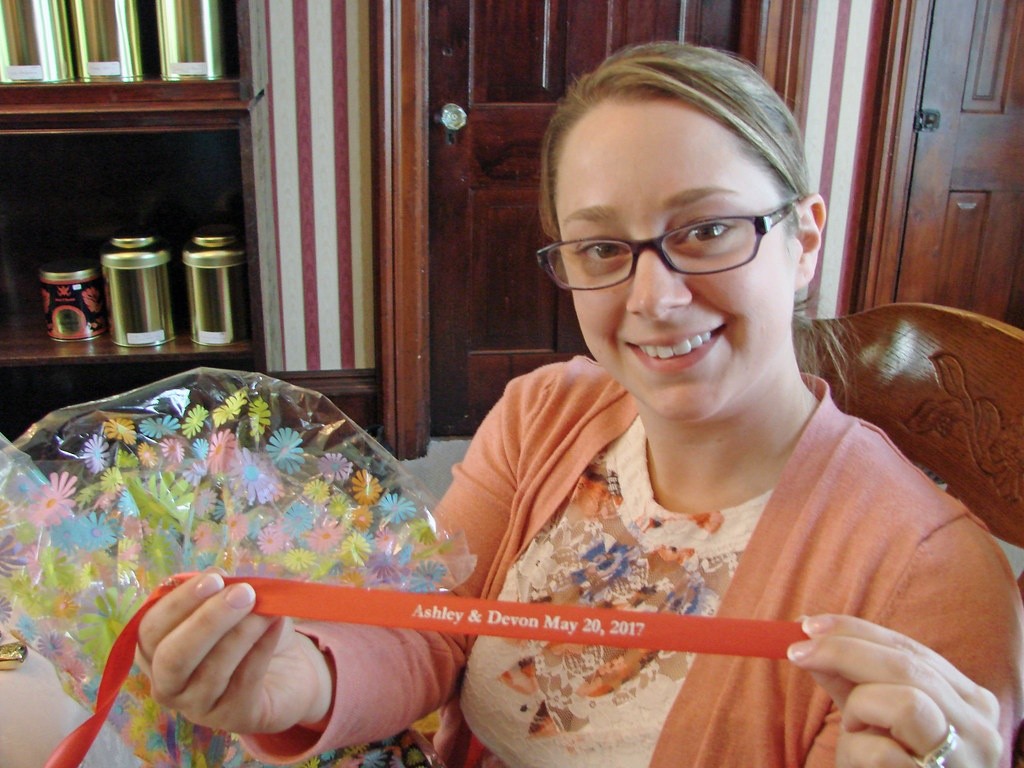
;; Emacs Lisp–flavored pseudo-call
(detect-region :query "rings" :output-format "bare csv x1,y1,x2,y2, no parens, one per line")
912,725,957,768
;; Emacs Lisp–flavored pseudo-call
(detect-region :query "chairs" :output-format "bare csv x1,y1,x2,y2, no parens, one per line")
795,303,1024,610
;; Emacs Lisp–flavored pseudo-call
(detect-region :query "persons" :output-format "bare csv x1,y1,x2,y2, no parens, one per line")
133,40,1024,768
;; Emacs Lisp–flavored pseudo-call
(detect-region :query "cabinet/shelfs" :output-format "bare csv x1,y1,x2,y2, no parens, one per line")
0,0,271,372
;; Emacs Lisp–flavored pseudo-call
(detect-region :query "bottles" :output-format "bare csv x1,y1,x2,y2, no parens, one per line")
39,259,99,341
182,225,248,347
100,236,176,348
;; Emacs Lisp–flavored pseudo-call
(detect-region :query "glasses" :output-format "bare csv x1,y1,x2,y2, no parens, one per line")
536,192,809,291
